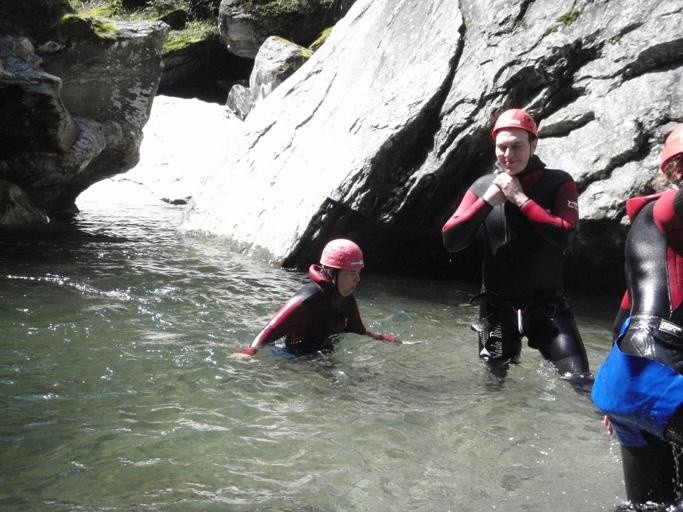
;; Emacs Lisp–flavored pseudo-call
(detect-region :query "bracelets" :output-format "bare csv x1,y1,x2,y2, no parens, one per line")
517,198,530,206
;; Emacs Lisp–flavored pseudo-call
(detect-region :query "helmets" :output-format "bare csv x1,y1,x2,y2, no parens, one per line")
493,109,538,137
661,125,682,172
319,239,364,270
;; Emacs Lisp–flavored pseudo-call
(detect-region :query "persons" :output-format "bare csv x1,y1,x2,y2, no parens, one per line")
442,107,594,400
651,183,682,257
589,125,682,512
227,238,424,386
